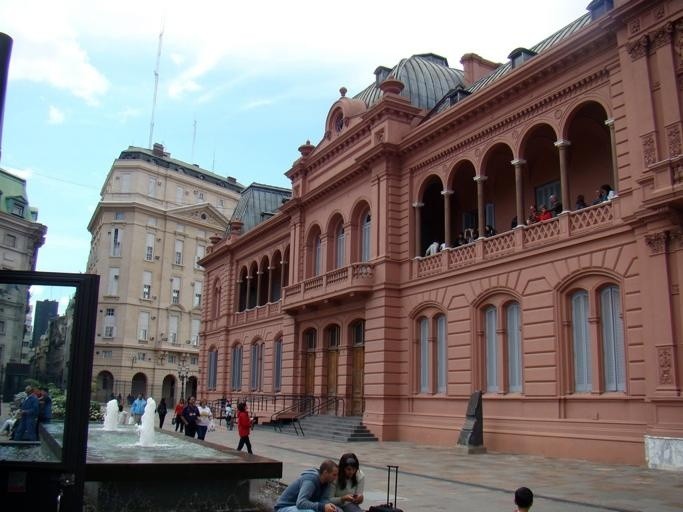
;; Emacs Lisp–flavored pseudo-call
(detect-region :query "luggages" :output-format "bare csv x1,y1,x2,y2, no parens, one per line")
368,465,402,512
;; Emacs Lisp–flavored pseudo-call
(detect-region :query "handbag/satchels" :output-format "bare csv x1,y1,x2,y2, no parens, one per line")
172,418,175,424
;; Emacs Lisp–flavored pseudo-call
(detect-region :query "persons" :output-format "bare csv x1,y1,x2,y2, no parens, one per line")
424,183,618,258
510,487,534,512
106,392,257,454
273,460,343,512
8,385,53,442
328,453,365,512
